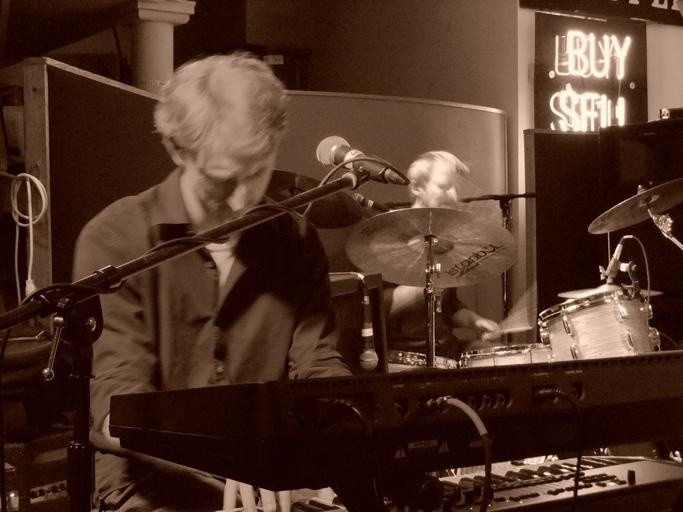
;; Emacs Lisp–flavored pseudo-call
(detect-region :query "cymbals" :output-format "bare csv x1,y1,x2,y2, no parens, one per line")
345,207,518,288
264,170,363,228
588,177,683,235
557,284,663,300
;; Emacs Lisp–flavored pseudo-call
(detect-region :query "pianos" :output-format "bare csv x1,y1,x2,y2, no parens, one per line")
109,350,683,492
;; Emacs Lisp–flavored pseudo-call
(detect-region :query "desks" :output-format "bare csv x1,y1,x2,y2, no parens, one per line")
328,270,390,375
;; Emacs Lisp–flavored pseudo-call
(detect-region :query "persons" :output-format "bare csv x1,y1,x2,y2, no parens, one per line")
72,53,353,511
382,149,500,362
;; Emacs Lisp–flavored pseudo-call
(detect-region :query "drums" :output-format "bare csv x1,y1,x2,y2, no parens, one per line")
456,343,554,369
387,350,457,373
538,285,661,363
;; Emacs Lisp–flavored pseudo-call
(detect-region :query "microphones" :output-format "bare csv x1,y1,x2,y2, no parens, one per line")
316,136,406,186
605,237,625,279
351,192,393,213
